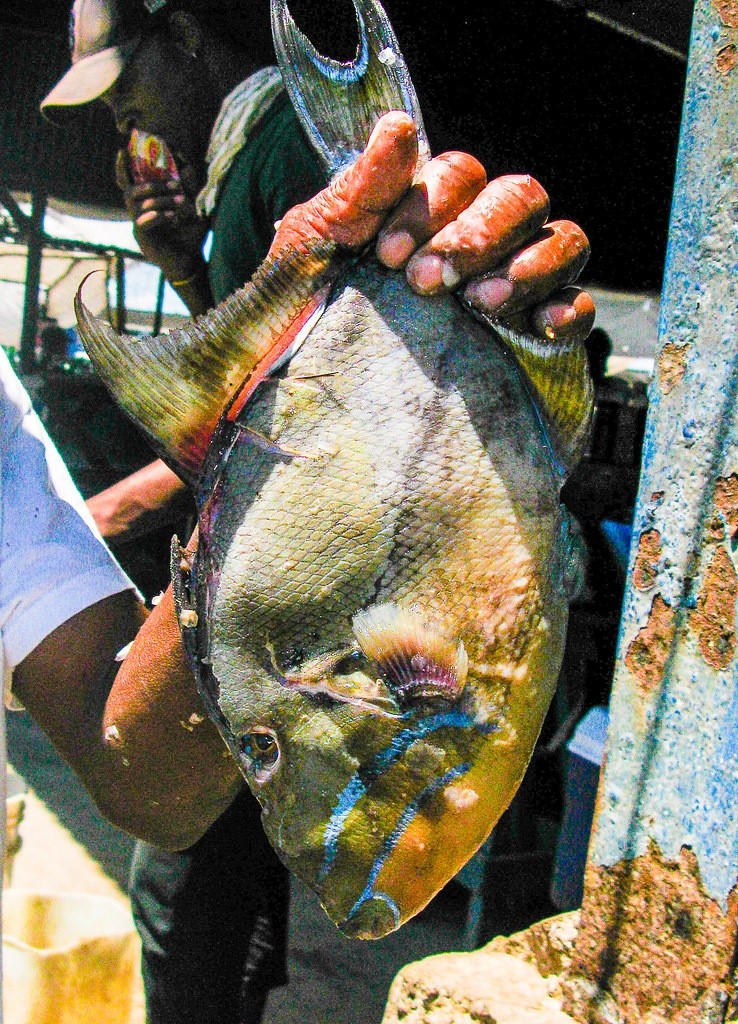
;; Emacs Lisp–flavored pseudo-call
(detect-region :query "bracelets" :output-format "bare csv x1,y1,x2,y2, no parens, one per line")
165,265,202,288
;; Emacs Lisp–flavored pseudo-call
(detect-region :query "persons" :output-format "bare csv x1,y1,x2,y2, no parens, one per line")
1,112,597,855
39,0,331,1024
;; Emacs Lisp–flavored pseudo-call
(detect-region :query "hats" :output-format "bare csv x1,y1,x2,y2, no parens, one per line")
39,0,204,136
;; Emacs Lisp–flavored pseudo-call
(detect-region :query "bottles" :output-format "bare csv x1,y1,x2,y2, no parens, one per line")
41,319,67,377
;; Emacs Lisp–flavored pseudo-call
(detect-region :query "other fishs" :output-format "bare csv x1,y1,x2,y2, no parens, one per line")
75,0,597,940
